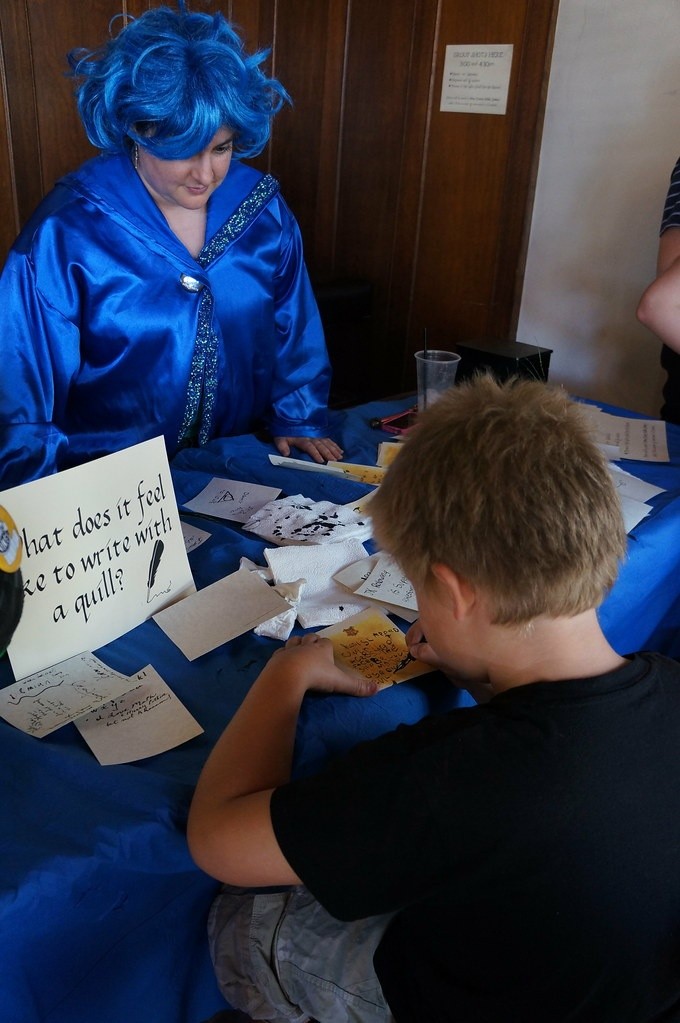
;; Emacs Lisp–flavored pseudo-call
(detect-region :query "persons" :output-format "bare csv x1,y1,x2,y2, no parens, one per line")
637,157,680,429
186,371,680,1023
0,0,345,495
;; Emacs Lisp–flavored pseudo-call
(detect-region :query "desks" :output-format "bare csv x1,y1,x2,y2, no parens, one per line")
0,383,680,1023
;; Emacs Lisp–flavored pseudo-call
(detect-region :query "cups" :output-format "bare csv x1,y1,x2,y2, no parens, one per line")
415,350,461,412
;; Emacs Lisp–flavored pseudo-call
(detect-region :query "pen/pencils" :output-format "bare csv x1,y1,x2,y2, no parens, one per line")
392,636,426,674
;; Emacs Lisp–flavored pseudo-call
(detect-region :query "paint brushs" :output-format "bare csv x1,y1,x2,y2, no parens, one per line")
421,326,428,411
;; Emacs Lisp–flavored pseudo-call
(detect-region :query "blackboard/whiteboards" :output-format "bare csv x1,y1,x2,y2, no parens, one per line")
510,1,680,419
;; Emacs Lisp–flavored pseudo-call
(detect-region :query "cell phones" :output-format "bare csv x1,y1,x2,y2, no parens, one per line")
381,411,418,435
